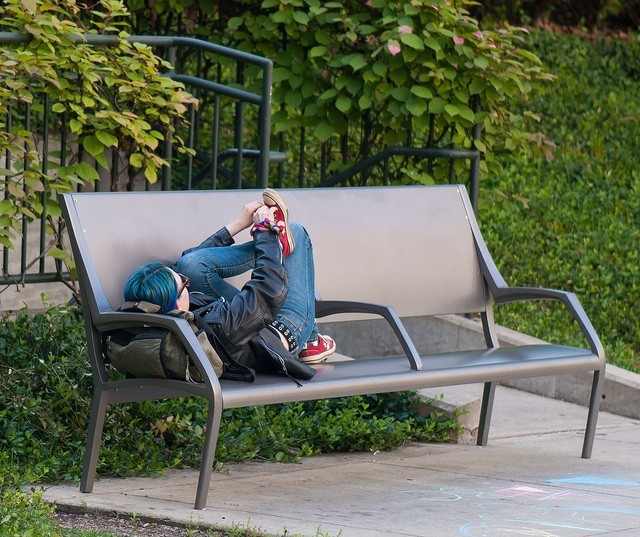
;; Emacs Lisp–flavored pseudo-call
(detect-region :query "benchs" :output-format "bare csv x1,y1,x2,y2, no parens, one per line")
59,183,605,509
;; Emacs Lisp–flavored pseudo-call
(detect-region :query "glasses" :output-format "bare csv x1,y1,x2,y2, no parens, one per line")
176,272,190,299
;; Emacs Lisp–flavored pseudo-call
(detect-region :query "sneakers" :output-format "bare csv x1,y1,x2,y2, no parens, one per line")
299,332,337,364
263,187,296,256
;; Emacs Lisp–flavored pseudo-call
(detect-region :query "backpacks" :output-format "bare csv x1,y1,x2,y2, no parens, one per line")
102,301,255,390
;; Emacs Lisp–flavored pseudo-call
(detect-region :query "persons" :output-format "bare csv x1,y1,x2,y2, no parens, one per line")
125,186,338,390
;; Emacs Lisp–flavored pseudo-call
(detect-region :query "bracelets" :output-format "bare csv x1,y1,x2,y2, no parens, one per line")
247,214,285,241
